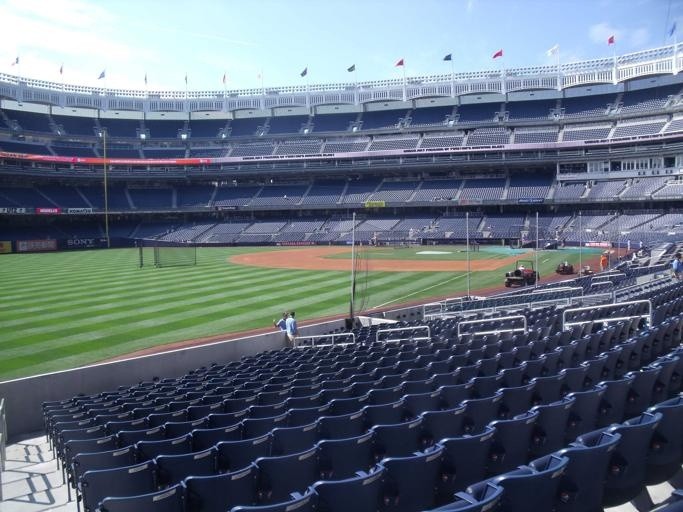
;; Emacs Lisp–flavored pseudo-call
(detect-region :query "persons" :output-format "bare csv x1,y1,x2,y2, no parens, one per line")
519,261,525,273
285,313,301,346
600,254,607,271
273,311,287,331
671,254,683,280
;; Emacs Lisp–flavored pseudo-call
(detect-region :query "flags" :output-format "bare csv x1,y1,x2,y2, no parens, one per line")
394,58,404,66
11,57,21,64
667,22,675,37
491,50,502,58
58,65,64,73
546,46,558,57
96,69,107,79
184,76,187,83
300,70,307,77
144,75,148,83
222,74,225,84
444,53,451,61
606,34,615,46
347,66,355,73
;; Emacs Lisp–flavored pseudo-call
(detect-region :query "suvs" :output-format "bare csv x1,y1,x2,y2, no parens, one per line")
504,259,539,287
